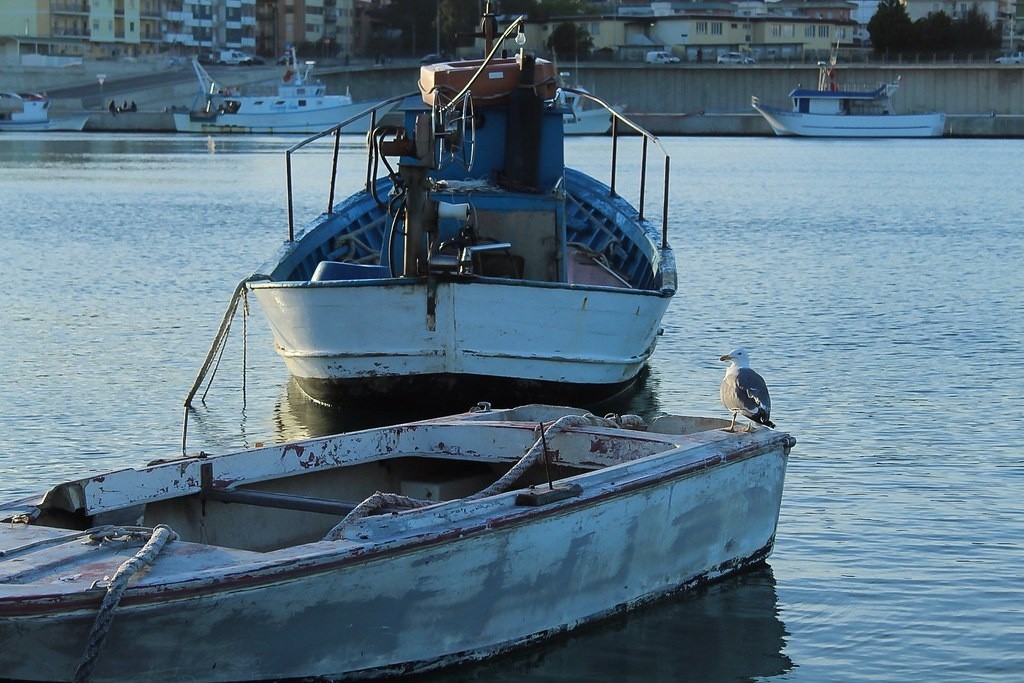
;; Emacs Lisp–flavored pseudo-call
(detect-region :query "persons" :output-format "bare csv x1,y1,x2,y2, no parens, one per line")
697,48,702,63
109,100,137,116
376,48,385,64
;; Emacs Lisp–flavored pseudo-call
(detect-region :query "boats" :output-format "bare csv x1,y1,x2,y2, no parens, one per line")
0,402,799,683
752,62,947,140
173,47,403,134
562,86,627,135
243,0,678,408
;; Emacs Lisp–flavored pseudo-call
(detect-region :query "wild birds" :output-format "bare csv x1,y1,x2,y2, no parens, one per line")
720,348,776,433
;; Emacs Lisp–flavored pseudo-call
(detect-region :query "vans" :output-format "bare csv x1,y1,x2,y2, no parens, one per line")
646,51,681,64
995,52,1024,64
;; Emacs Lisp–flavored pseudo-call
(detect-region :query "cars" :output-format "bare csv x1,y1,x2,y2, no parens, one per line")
0,92,51,115
277,55,298,65
718,53,755,64
220,50,252,65
